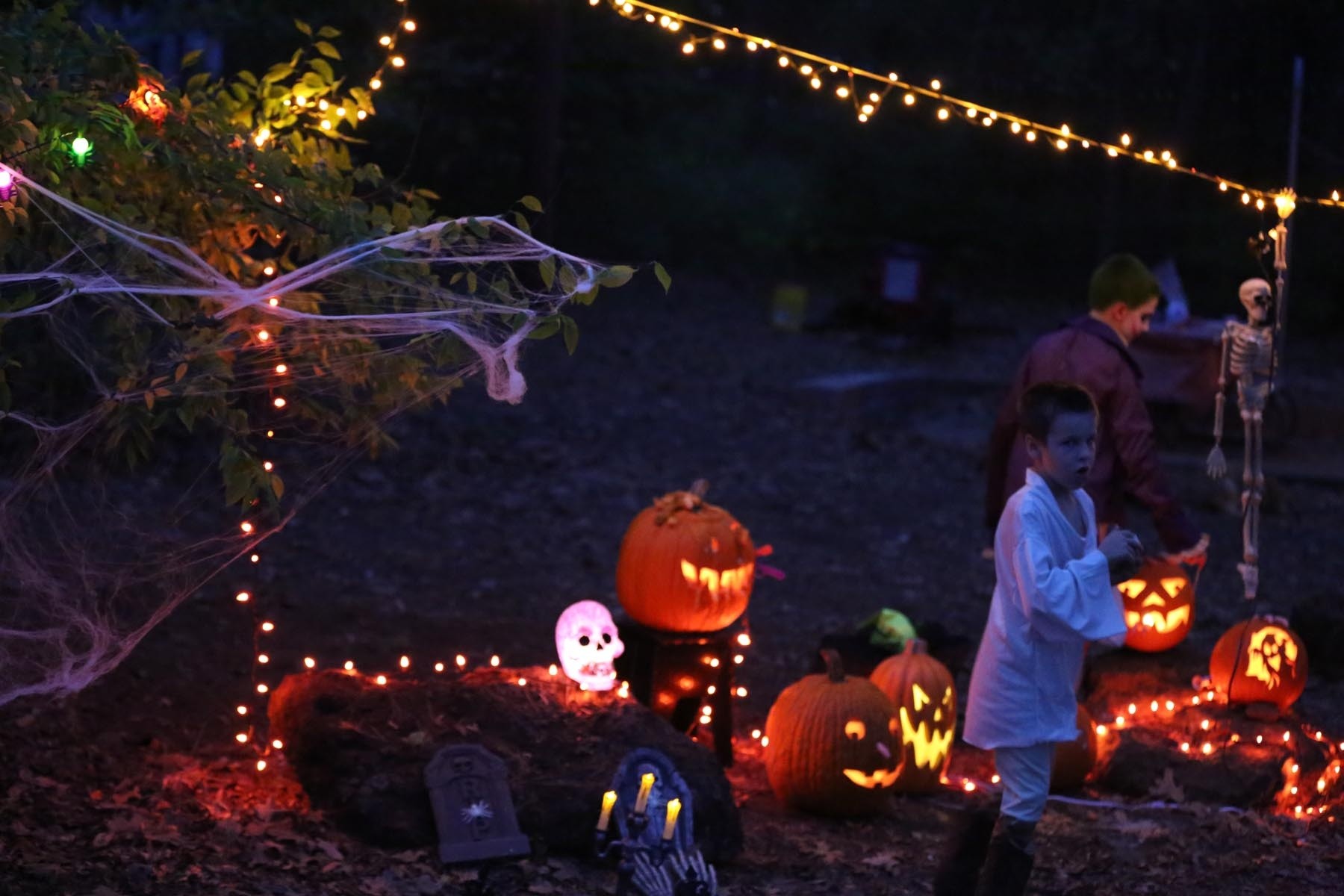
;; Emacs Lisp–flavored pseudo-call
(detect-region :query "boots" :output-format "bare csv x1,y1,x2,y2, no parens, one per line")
932,803,995,896
975,812,1038,895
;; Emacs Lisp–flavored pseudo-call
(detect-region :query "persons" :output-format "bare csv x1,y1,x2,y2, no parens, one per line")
981,262,1174,532
937,381,1145,894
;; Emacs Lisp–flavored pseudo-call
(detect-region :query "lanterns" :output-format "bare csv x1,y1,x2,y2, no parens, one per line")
766,649,905,812
1209,618,1308,711
617,490,756,632
1112,557,1195,650
869,637,958,784
